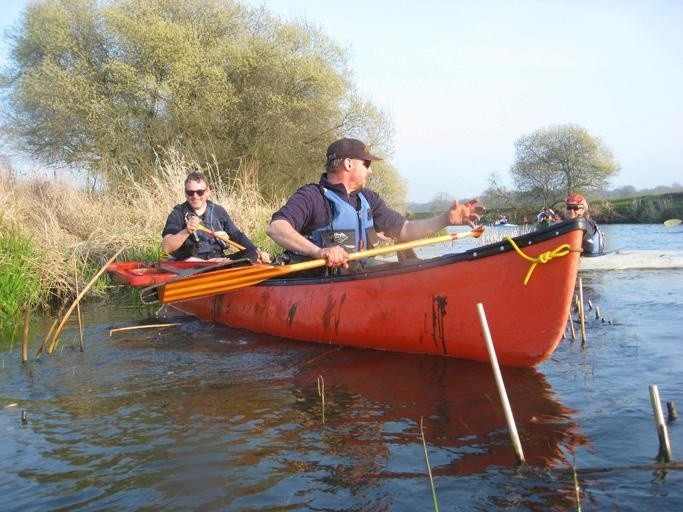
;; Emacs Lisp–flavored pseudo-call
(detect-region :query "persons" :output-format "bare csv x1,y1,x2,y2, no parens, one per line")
489,213,508,225
533,193,588,225
161,172,269,269
263,133,486,277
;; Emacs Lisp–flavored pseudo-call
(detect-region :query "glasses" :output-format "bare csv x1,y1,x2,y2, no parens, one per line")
351,157,371,168
185,188,206,196
566,206,583,210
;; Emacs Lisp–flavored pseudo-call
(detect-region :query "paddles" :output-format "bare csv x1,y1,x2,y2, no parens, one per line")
160,226,485,308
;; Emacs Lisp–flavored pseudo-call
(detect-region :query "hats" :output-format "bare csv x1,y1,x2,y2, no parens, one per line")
326,139,382,160
564,194,587,212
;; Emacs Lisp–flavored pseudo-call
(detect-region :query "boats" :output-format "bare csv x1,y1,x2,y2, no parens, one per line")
489,215,518,227
536,221,558,230
127,318,578,480
573,249,683,273
104,218,588,374
